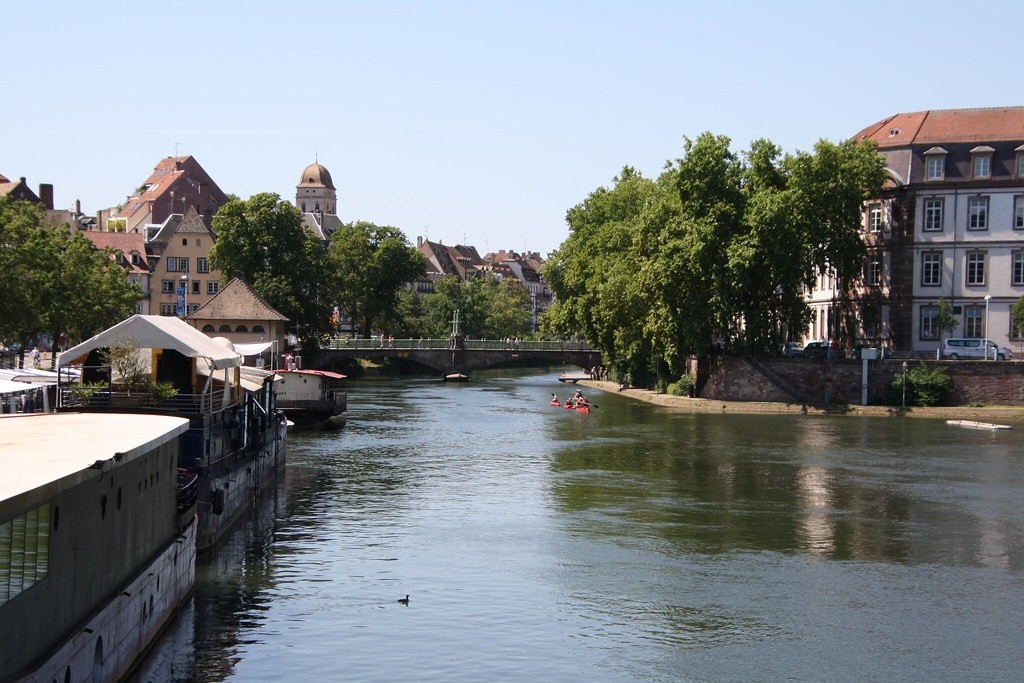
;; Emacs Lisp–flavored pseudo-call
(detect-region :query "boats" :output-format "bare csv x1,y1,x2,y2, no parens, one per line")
0,313,287,683
441,371,470,381
549,400,591,414
266,353,348,428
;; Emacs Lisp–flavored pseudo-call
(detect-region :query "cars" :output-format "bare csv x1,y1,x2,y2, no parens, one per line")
850,344,893,359
782,341,846,360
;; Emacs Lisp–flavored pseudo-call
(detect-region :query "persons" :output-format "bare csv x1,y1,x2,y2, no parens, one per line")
29,389,36,413
31,347,40,365
591,367,608,382
822,335,852,358
506,336,519,349
552,389,589,408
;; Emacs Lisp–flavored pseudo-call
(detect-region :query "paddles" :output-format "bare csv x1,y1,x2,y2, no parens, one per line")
590,404,599,408
551,392,560,403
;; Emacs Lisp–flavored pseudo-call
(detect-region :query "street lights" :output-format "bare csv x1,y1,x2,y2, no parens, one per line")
983,294,992,360
180,274,188,324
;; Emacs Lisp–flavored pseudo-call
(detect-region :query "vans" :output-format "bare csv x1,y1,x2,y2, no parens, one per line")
942,337,1013,361
